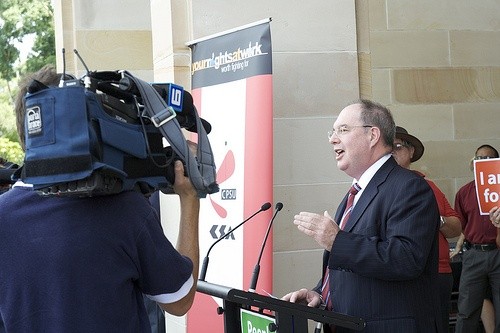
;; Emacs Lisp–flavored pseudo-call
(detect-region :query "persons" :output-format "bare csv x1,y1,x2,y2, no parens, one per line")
392,127,462,333
281,99,440,333
0,67,201,333
450,144,500,333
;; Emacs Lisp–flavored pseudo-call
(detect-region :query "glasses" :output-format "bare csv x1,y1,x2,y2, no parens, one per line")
475,156,496,160
328,124,373,139
394,142,409,150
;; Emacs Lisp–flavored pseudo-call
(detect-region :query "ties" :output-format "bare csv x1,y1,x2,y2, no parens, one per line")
319,182,362,312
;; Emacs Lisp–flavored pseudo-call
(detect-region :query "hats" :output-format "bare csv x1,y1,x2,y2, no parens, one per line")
394,126,424,163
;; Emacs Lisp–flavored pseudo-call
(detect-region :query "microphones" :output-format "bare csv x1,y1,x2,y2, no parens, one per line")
248,202,284,293
200,202,272,281
183,114,212,134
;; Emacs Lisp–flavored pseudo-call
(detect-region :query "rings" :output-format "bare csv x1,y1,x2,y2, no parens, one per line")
495,220,499,224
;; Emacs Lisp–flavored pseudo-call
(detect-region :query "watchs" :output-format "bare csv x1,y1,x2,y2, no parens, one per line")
440,216,446,228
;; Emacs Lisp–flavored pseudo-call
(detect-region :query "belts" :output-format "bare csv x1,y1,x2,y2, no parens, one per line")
471,242,497,252
